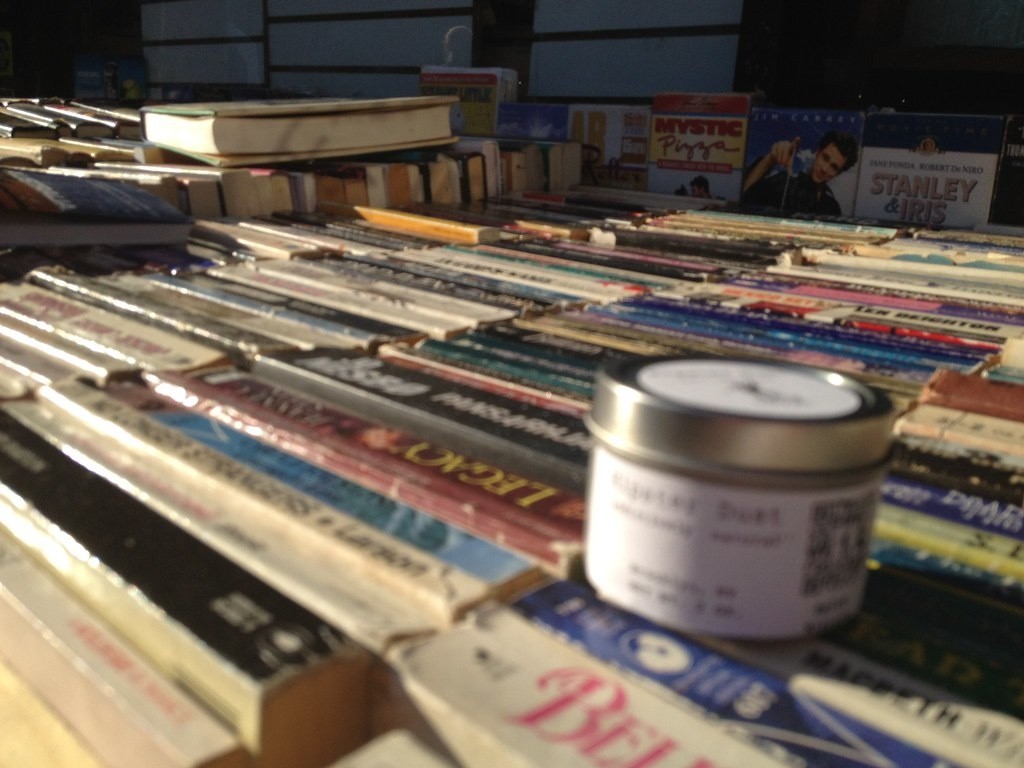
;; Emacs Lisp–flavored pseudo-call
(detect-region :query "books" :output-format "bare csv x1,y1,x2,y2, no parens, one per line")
1,66,1024,768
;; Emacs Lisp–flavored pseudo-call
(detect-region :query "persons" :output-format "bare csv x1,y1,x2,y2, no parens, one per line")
690,175,716,198
743,130,860,218
